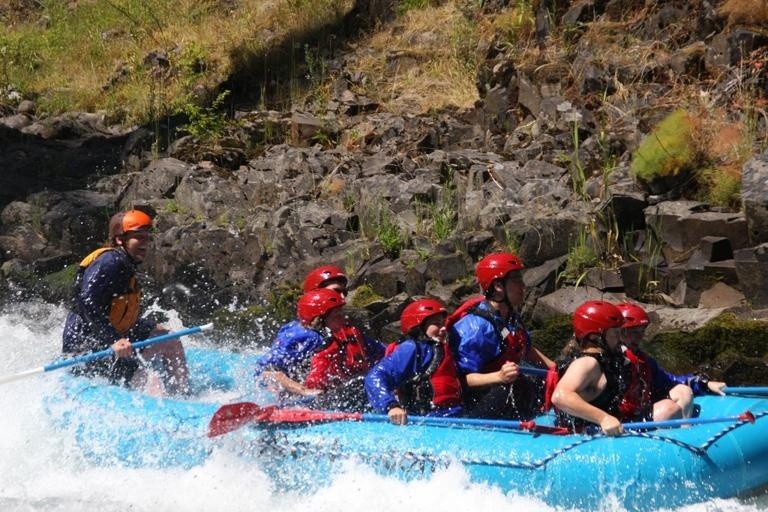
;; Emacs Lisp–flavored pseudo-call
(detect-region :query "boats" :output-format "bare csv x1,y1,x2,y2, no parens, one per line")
38,342,768,512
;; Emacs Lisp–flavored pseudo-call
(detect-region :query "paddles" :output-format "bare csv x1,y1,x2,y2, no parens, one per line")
257,408,535,429
207,396,316,438
536,410,754,434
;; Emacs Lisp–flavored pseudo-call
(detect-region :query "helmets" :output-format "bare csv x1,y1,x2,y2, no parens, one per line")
615,303,649,330
304,265,347,292
478,254,522,290
109,210,153,240
574,301,624,339
401,299,446,333
298,289,346,322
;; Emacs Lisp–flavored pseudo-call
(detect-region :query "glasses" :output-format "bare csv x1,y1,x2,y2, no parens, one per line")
124,232,152,241
424,317,445,327
505,270,522,282
334,288,347,296
601,328,621,337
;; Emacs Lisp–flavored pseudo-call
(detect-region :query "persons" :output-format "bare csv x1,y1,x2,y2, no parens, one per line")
618,301,726,429
303,267,385,360
447,251,557,420
62,210,190,397
550,299,680,437
254,287,364,413
364,298,462,425
